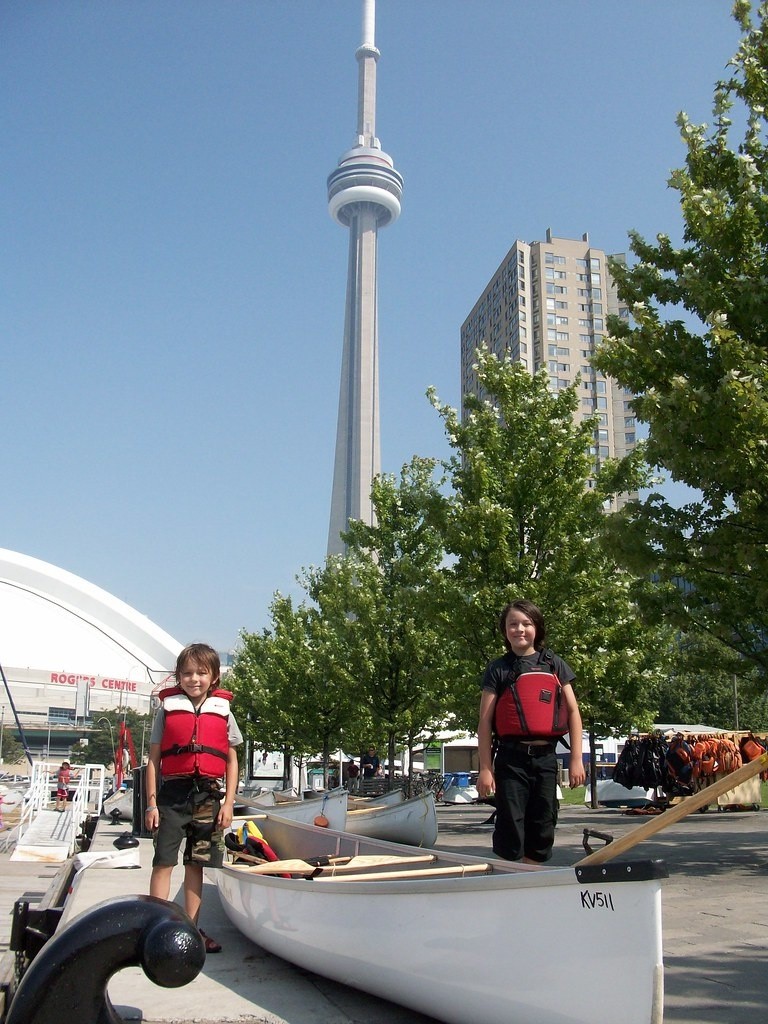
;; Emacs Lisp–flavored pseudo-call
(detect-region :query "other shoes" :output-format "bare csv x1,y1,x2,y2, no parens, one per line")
54,809,59,811
59,809,65,812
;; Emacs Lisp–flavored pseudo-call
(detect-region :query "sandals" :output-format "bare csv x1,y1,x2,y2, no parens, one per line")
199,930,222,953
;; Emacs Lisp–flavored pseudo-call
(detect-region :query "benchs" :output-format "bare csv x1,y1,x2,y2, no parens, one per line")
348,778,408,797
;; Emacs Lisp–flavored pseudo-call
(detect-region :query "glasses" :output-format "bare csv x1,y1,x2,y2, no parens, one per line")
369,750,373,751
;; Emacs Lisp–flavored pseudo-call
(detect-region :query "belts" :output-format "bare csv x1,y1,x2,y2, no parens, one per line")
517,742,553,756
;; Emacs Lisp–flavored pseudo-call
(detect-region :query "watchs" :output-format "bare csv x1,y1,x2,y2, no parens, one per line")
145,806,157,812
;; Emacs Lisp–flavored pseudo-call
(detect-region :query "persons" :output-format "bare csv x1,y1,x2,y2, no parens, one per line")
346,759,359,795
476,599,585,866
360,746,382,780
145,644,244,953
0,791,15,829
42,759,83,812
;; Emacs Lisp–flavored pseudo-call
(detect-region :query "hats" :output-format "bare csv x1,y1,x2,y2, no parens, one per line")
62,759,71,767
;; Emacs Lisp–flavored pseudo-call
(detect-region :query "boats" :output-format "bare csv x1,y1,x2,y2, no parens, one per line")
211,802,667,1024
343,790,440,849
259,786,352,832
102,788,135,821
223,785,404,809
